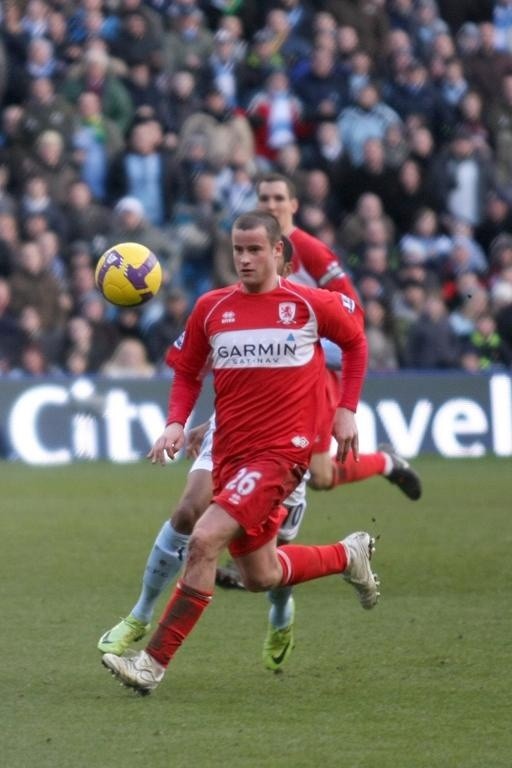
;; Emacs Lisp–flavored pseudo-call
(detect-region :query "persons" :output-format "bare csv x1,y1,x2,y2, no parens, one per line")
205,173,425,592
100,209,382,694
96,229,311,678
2,0,511,379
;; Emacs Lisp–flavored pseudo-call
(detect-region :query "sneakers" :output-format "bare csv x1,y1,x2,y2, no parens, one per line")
377,442,421,502
344,531,379,609
96,615,153,655
103,651,166,696
262,598,296,672
214,567,246,591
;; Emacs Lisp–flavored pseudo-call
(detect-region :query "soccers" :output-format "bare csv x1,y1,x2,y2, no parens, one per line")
94,242,161,305
